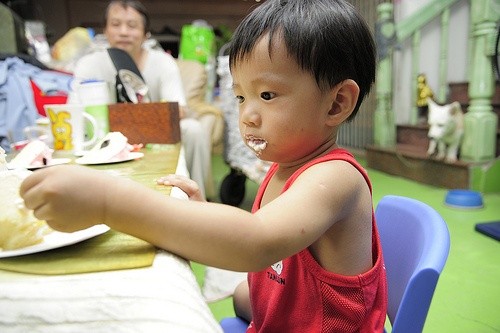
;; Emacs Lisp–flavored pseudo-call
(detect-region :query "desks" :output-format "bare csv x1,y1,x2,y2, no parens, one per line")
0,135,223,333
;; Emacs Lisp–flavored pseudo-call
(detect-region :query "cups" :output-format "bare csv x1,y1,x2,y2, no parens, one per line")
42,103,100,157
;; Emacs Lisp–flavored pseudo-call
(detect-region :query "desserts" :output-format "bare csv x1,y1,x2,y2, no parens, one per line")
84,131,130,161
0,141,55,252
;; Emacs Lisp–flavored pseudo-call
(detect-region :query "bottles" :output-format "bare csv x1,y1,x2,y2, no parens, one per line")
78,79,110,144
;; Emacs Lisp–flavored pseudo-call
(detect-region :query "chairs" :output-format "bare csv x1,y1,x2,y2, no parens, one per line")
216,194,451,333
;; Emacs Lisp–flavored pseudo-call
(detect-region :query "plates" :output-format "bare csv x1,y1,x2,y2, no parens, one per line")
7,157,73,171
0,206,110,261
74,145,143,165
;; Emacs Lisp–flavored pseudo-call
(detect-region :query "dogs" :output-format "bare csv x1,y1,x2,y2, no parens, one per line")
425,96,464,164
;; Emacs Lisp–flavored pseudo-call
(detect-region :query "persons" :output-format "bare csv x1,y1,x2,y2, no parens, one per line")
20,0,387,333
67,0,210,201
